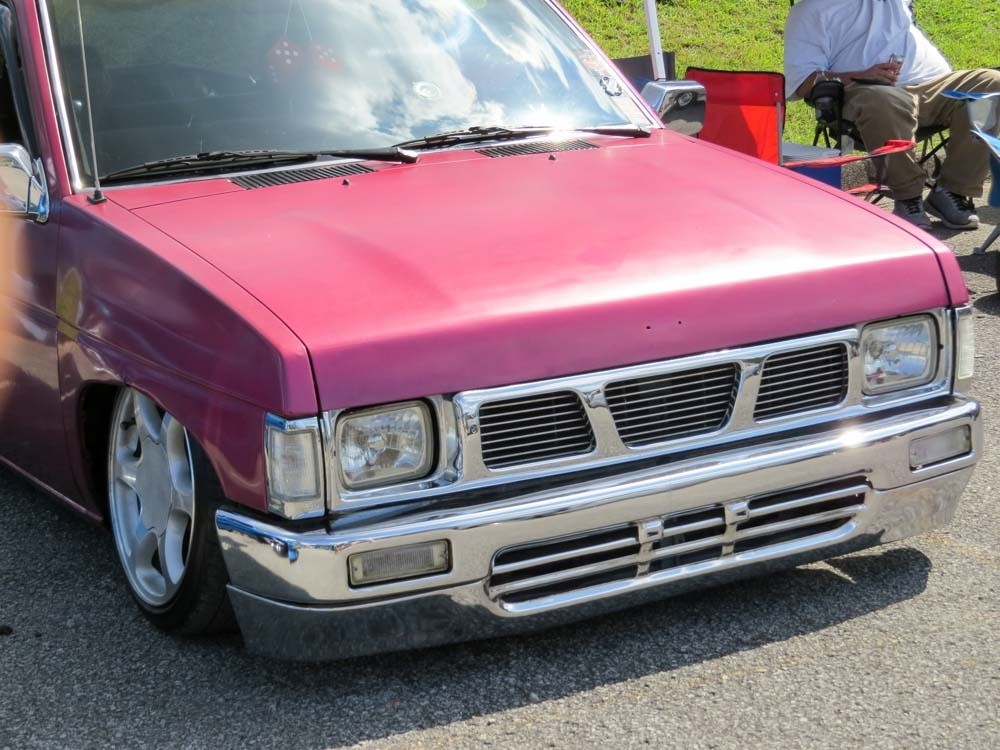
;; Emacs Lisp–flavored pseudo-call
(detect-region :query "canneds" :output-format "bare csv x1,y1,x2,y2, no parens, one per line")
887,52,905,75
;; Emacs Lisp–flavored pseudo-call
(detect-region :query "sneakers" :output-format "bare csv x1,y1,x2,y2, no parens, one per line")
892,197,932,229
922,184,980,229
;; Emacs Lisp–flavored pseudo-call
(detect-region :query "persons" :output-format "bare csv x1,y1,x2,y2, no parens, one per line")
782,0,1000,230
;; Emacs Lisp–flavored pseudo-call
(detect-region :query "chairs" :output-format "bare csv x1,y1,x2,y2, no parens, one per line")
803,80,951,199
685,65,917,204
938,89,1000,253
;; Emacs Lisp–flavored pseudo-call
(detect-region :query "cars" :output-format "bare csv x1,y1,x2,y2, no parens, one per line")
0,0,985,666
